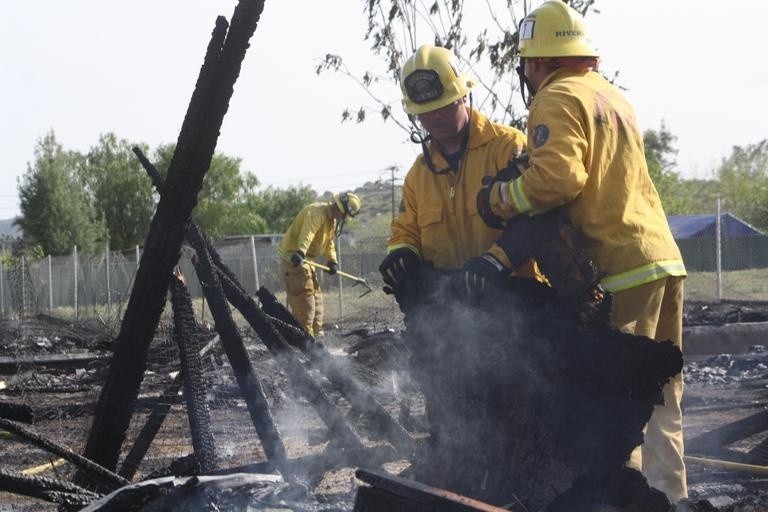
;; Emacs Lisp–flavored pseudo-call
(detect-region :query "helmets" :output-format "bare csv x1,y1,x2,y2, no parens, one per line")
331,191,362,219
400,45,469,115
515,0,599,59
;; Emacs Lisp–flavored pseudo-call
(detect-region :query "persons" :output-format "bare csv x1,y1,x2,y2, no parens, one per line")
475,1,688,500
276,191,363,336
375,38,550,303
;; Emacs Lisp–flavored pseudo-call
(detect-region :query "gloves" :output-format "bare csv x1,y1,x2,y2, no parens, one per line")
379,247,418,288
290,250,305,267
327,261,336,275
477,176,504,229
461,252,511,297
